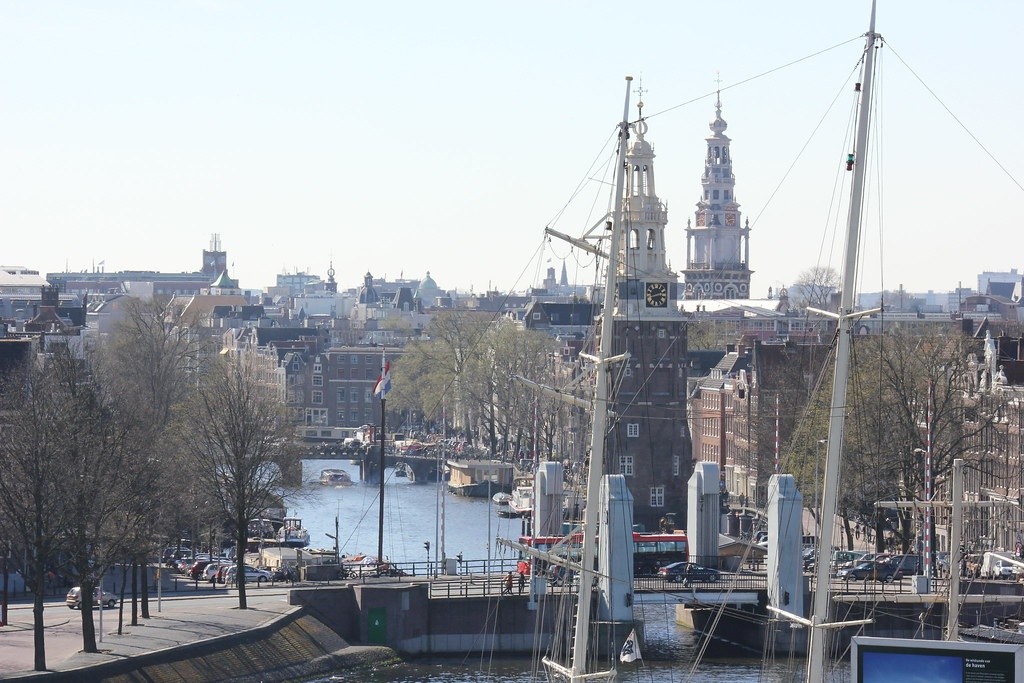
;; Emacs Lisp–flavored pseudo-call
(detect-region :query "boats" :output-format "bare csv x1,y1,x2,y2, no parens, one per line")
507,477,534,517
318,469,353,487
492,491,512,505
446,458,515,496
251,547,388,578
276,516,310,547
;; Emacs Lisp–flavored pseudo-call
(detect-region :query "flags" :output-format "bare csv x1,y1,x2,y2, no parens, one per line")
391,359,409,414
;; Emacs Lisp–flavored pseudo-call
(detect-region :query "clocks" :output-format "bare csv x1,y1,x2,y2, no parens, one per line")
645,282,667,307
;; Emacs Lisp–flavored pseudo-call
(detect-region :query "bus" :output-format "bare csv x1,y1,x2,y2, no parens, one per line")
516,533,690,579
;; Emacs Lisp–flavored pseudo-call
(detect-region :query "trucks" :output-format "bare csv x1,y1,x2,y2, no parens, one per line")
981,552,1011,580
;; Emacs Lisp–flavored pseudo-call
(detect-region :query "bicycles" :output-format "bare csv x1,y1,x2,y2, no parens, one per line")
336,567,358,580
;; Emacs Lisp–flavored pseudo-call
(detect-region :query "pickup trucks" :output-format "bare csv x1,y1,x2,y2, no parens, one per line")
992,560,1018,580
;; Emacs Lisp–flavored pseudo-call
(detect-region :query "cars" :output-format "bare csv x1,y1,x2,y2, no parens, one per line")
226,565,270,584
162,546,248,583
657,562,721,584
547,564,598,587
839,563,904,584
753,530,981,580
66,587,118,610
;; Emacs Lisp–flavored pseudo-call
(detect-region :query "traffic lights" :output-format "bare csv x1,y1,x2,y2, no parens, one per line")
625,594,632,610
424,541,430,551
456,552,463,563
785,591,789,606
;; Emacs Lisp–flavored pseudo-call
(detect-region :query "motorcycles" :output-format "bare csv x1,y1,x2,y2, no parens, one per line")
273,560,297,583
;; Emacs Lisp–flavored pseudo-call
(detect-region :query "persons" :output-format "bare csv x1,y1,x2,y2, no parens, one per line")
684,561,694,588
518,571,527,593
854,522,862,540
502,571,513,593
739,493,745,507
